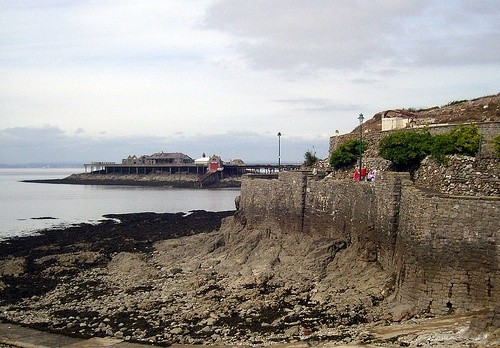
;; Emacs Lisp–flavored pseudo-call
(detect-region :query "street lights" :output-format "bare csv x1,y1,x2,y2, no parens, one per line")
358,113,364,181
277,132,281,172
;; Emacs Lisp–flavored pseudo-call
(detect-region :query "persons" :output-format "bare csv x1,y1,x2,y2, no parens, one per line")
354,164,376,182
